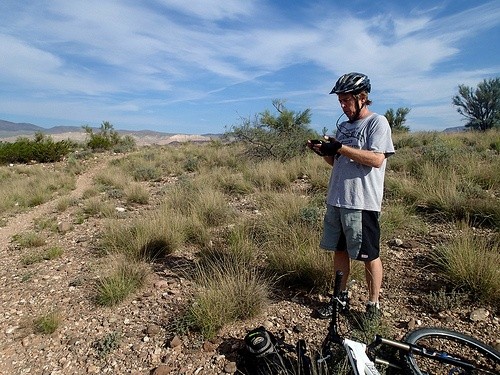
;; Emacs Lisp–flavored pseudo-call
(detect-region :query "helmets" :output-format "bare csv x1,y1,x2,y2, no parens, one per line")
329,72,371,95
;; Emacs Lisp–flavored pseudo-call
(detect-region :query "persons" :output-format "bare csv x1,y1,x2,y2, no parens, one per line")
307,72,395,331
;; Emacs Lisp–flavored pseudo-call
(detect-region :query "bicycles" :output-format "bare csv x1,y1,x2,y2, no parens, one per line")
317,270,500,375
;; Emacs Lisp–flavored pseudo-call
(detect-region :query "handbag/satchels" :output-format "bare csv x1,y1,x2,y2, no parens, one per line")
243,327,293,375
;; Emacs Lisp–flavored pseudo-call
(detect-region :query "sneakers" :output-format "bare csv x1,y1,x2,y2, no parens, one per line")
362,304,383,330
313,297,350,318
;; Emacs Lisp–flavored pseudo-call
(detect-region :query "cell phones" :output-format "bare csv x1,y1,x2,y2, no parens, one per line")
310,139,319,144
